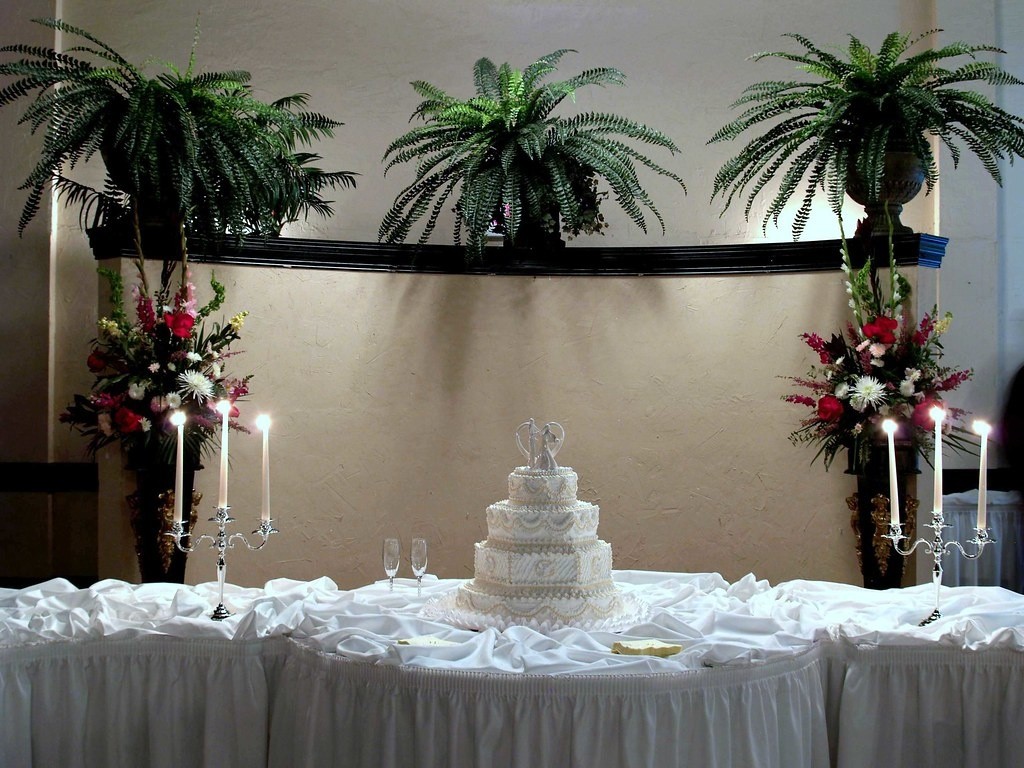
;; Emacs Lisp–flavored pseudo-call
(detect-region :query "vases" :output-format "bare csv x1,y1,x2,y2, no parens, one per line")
848,442,919,469
136,467,193,495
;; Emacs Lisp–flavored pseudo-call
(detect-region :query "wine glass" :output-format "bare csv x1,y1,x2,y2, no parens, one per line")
382,538,401,592
410,537,428,595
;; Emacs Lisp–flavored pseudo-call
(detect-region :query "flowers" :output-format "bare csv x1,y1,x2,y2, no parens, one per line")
774,200,997,471
58,197,258,470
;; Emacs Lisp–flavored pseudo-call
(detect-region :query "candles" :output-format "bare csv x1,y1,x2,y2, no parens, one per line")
174,425,183,522
218,413,228,507
888,433,900,524
977,436,987,529
934,421,942,513
261,429,270,520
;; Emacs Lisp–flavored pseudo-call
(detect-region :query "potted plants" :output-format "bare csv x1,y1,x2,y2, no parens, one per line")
377,47,688,264
704,28,1024,240
0,17,363,247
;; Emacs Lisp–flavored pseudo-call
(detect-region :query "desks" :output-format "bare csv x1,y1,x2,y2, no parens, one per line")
0,584,1024,768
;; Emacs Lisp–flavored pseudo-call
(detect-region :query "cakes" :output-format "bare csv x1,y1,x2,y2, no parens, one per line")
455,417,623,626
